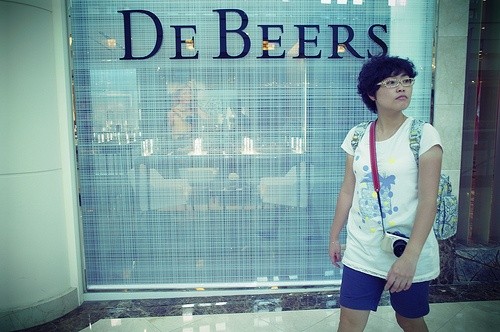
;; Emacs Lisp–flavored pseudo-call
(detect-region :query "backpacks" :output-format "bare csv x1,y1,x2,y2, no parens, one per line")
351,119,458,241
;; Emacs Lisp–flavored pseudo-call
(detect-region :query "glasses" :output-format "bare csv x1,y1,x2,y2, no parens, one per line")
377,78,416,88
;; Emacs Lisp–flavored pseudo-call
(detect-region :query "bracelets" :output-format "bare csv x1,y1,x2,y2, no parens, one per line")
330,241,339,244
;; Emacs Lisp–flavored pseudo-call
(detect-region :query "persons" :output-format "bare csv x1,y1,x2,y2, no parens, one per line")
226,101,251,178
168,85,206,140
328,57,444,332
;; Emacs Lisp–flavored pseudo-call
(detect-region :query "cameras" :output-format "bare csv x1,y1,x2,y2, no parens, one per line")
381,229,410,259
